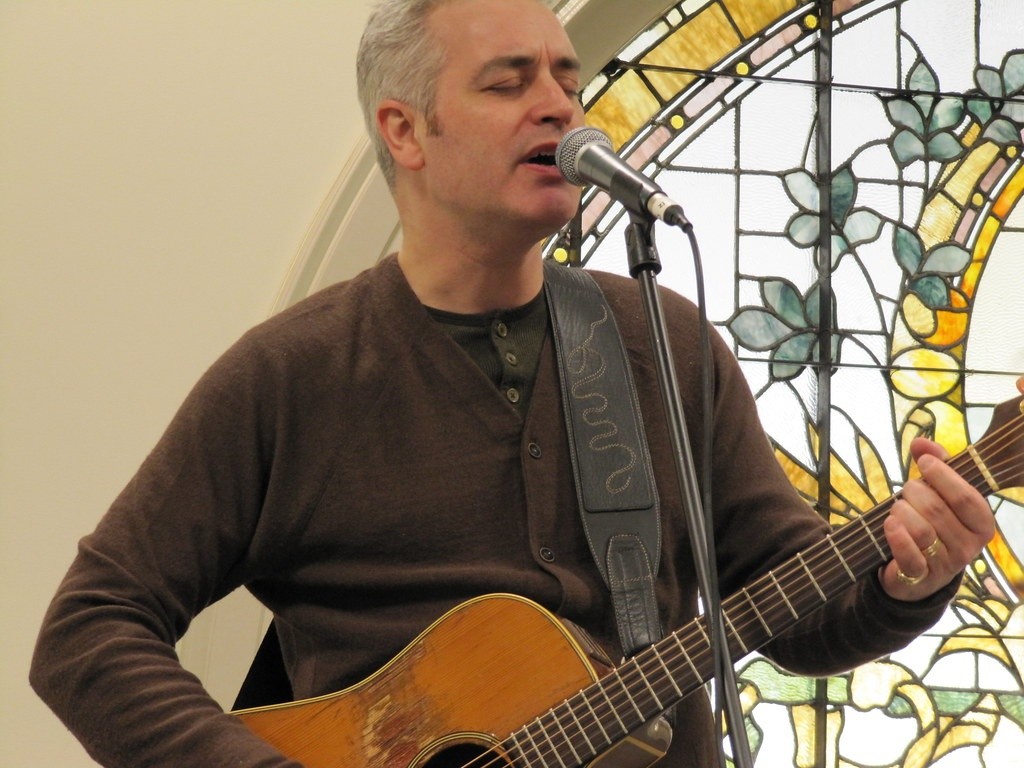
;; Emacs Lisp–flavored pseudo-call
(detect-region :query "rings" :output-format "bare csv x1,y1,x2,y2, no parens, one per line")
896,568,928,585
920,536,942,559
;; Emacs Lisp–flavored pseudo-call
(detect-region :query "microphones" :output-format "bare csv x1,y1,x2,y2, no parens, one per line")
553,123,691,235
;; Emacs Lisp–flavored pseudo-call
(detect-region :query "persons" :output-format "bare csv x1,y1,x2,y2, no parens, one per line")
25,0,997,768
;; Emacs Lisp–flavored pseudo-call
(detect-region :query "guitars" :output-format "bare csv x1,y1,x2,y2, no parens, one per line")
221,376,1024,768
